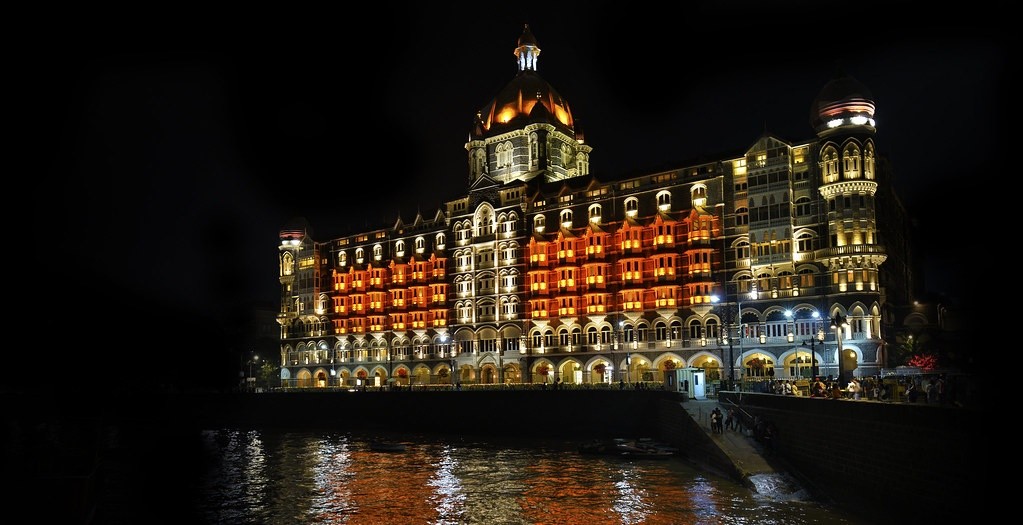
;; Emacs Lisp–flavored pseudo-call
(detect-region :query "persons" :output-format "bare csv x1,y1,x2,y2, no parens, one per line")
726,405,734,430
715,407,723,428
714,410,724,434
734,406,743,432
542,381,547,390
456,379,462,391
552,376,560,391
560,381,565,391
718,375,952,405
710,410,717,434
620,379,625,390
635,381,649,391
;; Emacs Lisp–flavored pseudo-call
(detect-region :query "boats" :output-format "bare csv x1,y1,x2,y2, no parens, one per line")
591,436,678,461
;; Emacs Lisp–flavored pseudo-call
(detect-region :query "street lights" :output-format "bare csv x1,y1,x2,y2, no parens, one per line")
830,312,848,388
711,284,758,391
